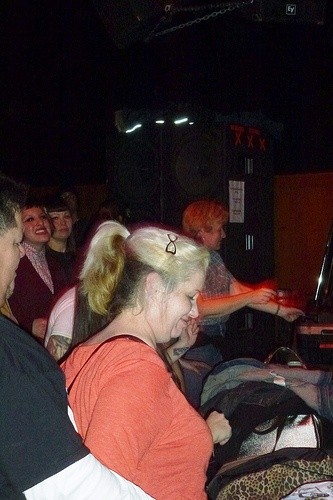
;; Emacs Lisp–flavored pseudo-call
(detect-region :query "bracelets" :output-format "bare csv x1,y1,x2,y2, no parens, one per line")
274,302,283,318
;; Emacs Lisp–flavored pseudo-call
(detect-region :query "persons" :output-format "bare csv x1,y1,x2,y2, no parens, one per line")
0,173,157,500
10,191,73,351
55,221,215,500
170,356,213,394
174,197,307,413
40,197,84,294
42,297,200,396
